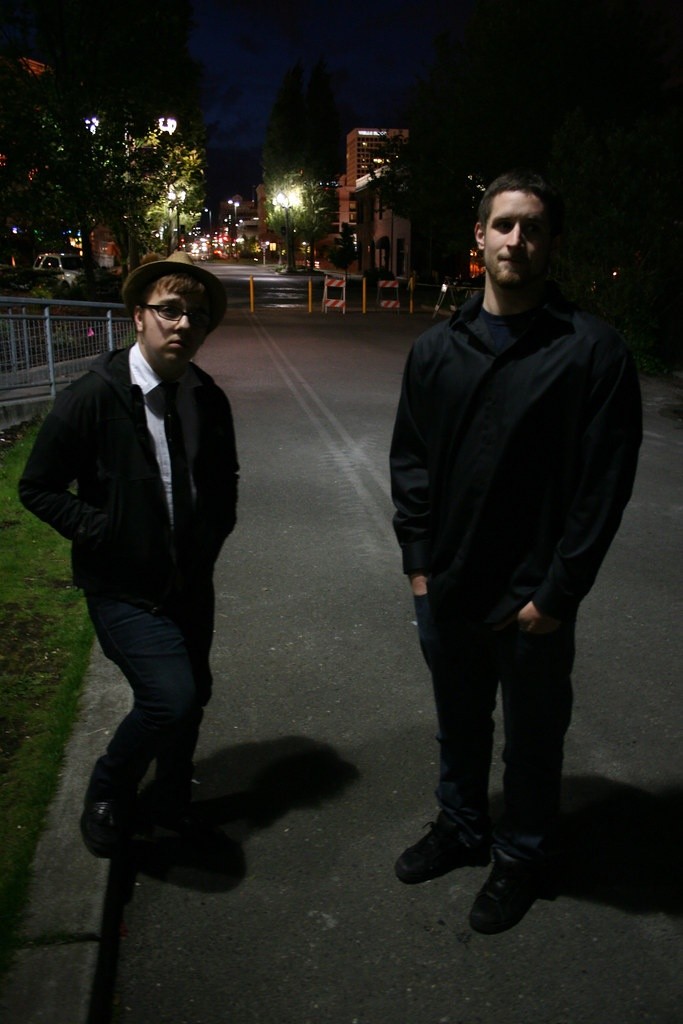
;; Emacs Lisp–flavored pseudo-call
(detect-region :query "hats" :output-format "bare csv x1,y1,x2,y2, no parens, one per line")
121,251,227,337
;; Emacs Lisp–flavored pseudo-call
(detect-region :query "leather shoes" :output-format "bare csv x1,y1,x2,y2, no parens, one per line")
156,812,209,834
80,792,122,858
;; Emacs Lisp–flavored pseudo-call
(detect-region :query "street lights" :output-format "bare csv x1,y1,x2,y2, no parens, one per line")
270,192,297,274
227,198,242,226
204,209,213,236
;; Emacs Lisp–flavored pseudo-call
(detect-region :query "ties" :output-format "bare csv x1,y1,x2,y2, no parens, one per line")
159,381,192,549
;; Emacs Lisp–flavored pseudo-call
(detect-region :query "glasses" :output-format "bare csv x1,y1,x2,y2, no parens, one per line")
139,304,212,327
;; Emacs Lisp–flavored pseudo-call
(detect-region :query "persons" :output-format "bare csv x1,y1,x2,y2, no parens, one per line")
388,167,645,934
16,249,241,858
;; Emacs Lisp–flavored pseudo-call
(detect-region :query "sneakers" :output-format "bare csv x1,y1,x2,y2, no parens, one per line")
469,847,537,934
394,811,466,884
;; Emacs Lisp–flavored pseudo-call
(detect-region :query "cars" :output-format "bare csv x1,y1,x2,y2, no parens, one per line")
33,253,104,295
